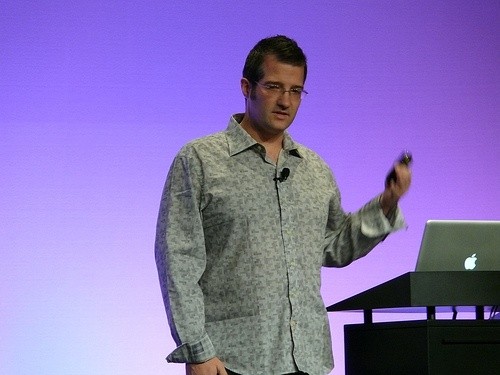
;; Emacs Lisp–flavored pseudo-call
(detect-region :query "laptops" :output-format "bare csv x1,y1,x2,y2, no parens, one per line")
414,220,500,271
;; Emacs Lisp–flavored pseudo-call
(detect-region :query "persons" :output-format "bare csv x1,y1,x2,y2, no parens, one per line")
154,35,413,375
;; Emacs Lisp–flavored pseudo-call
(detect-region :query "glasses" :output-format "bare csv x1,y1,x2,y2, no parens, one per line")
244,75,308,100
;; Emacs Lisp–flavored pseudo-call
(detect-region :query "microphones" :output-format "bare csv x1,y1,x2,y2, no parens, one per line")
282,168,290,180
